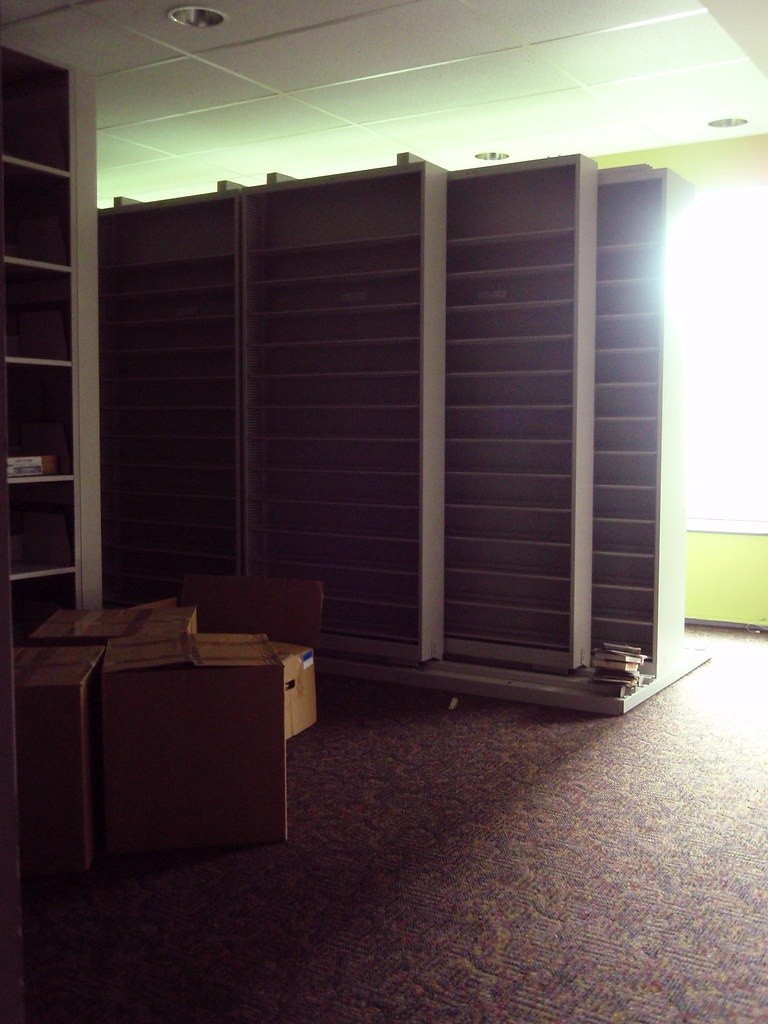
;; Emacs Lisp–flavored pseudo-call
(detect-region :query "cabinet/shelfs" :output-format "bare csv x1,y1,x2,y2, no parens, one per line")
0,41,657,715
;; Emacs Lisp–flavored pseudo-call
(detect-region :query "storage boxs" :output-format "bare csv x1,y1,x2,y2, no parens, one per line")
12,572,327,879
7,454,57,476
